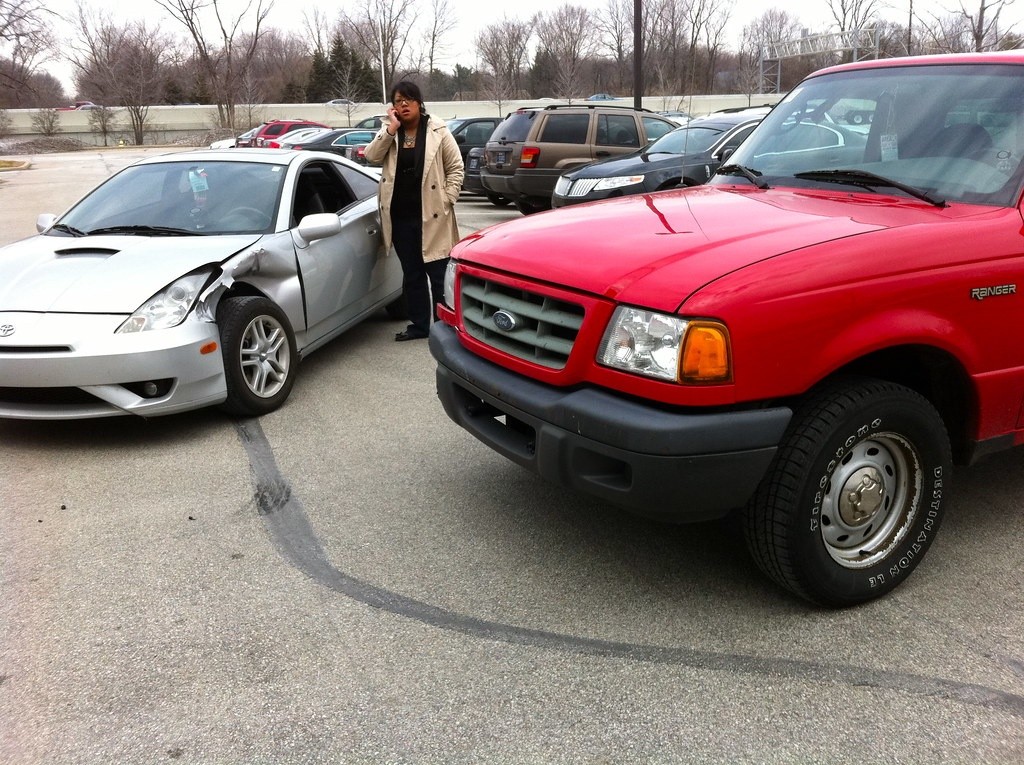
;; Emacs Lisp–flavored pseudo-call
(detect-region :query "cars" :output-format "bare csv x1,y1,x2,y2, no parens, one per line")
208,103,775,217
1,148,406,419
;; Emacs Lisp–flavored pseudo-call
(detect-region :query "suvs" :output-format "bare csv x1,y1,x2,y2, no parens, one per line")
426,48,1024,613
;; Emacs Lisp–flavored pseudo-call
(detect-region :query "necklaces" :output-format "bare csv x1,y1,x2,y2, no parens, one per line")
404,134,415,145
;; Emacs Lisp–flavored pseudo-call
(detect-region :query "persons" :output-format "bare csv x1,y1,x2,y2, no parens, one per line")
365,81,464,342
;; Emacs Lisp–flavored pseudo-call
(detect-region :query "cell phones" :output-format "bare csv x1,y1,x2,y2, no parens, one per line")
395,112,401,122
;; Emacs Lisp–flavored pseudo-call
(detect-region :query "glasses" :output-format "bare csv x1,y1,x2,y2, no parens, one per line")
393,96,414,105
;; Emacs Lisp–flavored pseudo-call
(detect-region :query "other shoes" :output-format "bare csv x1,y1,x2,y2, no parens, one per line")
395,329,428,341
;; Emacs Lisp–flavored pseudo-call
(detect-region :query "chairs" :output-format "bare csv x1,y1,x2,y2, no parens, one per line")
616,129,633,144
921,122,990,160
288,173,333,220
174,172,226,226
598,128,606,143
